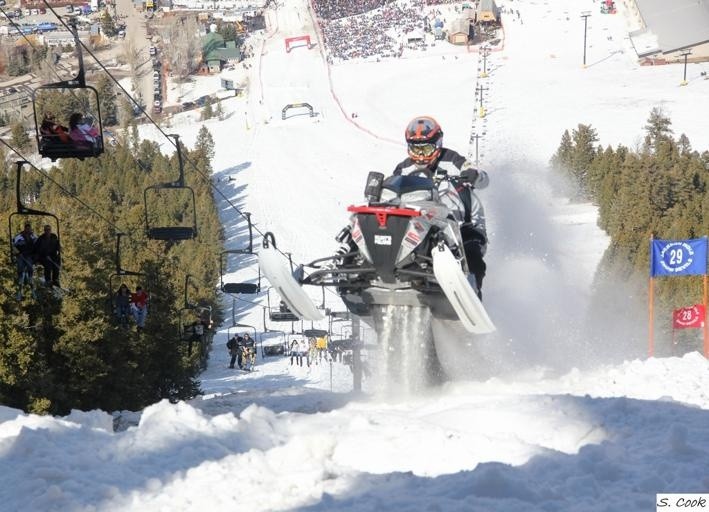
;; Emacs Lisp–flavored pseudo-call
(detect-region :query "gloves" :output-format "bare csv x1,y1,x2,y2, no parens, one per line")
460,168,479,188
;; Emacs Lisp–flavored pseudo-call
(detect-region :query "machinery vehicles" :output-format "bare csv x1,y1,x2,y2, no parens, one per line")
146,0,154,18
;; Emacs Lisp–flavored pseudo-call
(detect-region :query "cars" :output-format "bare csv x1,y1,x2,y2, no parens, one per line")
144,31,160,43
0,0,126,37
133,45,161,116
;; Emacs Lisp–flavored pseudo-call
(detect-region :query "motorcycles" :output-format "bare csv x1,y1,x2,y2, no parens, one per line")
255,172,500,341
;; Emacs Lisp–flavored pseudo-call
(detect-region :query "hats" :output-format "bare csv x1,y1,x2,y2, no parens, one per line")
43,111,56,120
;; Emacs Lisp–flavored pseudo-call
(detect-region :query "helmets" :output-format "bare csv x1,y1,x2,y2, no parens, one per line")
404,116,444,166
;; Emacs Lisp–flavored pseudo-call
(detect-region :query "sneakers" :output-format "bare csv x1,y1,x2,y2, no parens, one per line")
227,362,255,372
290,362,329,368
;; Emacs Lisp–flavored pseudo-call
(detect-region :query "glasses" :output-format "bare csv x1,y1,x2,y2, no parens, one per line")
49,116,56,120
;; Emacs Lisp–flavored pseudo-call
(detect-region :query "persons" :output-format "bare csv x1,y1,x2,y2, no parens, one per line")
114,284,132,324
227,334,243,370
290,334,346,366
69,113,99,151
14,224,38,301
241,333,256,372
394,116,490,303
308,0,523,64
131,286,150,329
36,224,60,290
186,315,213,357
40,111,69,151
